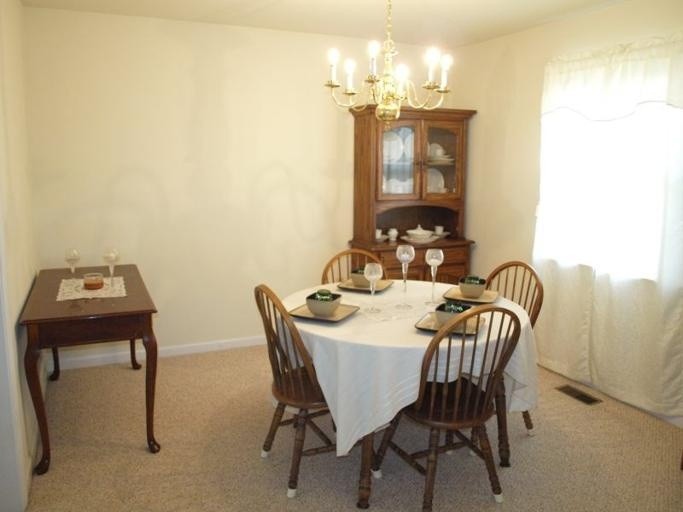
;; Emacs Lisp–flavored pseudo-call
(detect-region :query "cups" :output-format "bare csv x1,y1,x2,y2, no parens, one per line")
387,228,399,241
466,274,480,286
435,226,444,233
445,299,463,313
357,266,364,274
315,290,333,301
84,273,103,290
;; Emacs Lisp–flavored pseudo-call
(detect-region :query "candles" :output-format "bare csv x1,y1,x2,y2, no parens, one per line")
425,46,437,81
367,41,378,76
329,48,338,83
442,53,449,87
344,58,353,91
397,65,407,94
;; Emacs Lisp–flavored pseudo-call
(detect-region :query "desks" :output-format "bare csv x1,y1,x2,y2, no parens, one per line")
20,264,160,474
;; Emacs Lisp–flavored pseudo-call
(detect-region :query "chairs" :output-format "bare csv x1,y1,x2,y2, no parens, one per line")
377,305,520,512
321,249,385,286
464,261,542,455
254,286,380,497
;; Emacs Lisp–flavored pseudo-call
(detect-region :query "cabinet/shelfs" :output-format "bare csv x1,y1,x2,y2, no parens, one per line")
352,246,470,284
350,105,477,241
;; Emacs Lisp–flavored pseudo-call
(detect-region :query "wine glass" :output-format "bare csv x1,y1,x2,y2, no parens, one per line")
103,248,121,296
365,263,380,314
65,248,81,286
425,249,445,305
394,245,415,311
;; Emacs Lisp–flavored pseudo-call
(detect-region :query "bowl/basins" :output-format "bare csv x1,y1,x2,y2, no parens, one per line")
433,232,450,238
459,277,487,297
435,304,471,331
350,270,370,287
306,293,342,317
400,236,442,246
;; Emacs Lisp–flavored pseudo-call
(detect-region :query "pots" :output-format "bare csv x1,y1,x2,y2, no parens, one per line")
405,224,434,240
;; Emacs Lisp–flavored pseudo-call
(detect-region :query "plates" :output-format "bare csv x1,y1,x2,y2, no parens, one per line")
375,235,389,242
427,169,444,193
404,127,415,161
382,132,404,163
427,143,455,163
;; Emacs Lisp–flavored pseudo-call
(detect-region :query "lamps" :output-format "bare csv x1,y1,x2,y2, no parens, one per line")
324,0,453,130
